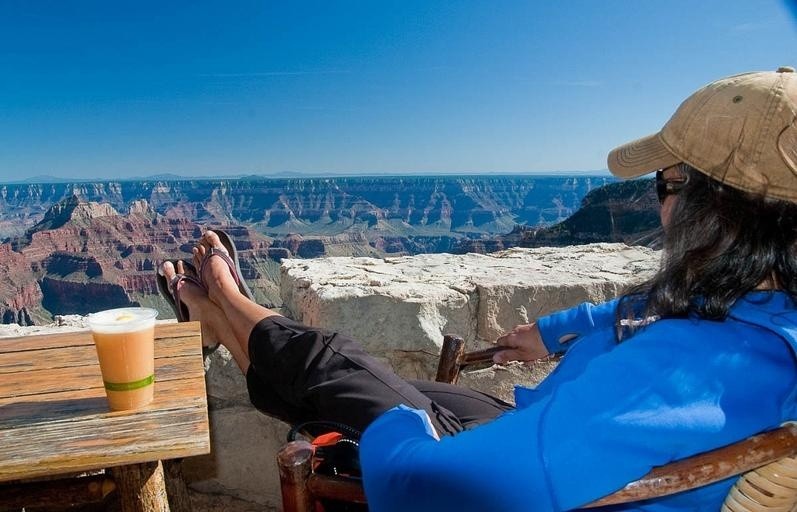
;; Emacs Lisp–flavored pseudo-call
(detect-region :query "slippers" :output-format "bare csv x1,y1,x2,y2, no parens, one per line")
198,228,257,315
156,258,221,356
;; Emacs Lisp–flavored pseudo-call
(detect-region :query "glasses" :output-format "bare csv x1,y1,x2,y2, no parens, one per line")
654,170,687,205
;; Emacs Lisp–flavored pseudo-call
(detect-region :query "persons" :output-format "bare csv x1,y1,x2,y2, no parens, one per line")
157,66,797,512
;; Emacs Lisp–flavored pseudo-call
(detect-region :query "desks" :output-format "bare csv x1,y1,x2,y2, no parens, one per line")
0,320,211,512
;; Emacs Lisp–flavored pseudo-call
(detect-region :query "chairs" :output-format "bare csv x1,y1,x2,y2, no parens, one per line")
278,333,797,512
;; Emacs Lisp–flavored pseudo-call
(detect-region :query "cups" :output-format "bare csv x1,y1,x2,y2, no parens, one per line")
86,306,158,412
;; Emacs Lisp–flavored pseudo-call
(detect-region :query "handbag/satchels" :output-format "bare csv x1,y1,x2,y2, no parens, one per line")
315,435,370,512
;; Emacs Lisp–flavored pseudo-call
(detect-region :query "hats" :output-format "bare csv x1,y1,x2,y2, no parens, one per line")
607,66,797,205
